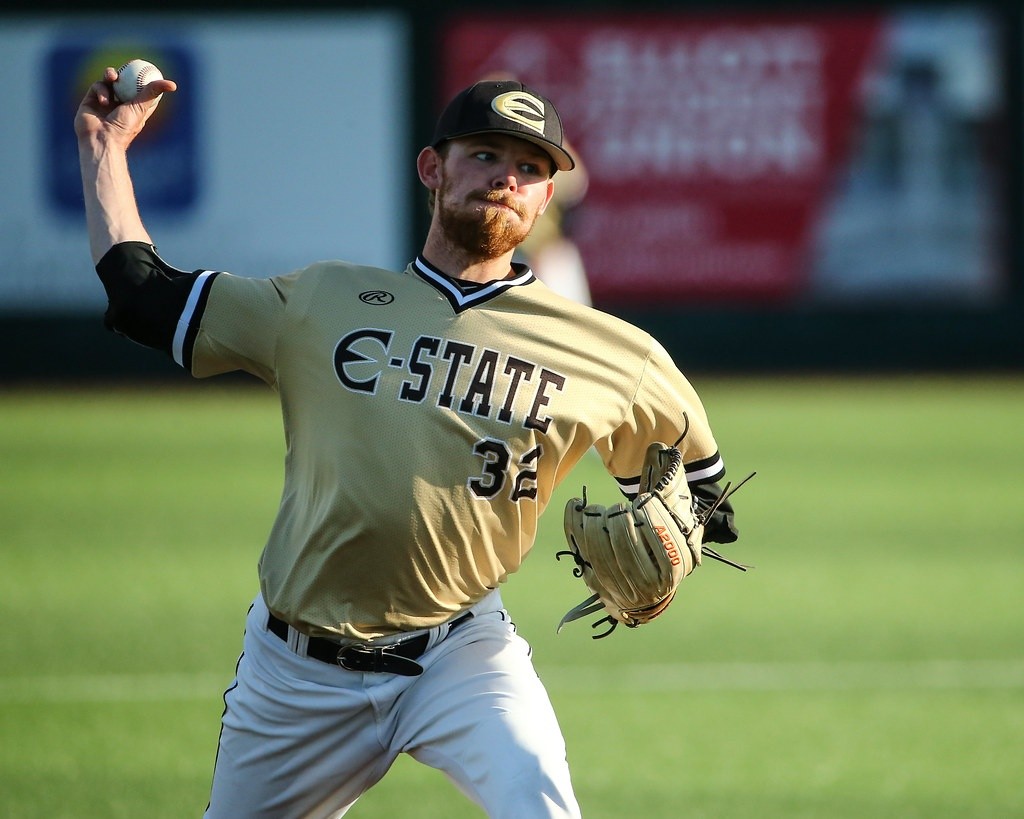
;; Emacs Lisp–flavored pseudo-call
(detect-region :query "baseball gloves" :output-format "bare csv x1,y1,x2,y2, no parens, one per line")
563,442,708,628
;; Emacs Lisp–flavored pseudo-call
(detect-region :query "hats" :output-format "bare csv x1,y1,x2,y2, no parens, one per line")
433,80,576,172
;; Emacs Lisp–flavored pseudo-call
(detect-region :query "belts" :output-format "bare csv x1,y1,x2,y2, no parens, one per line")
266,611,475,676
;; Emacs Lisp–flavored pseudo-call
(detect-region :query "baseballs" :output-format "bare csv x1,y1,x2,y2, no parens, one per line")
113,59,165,103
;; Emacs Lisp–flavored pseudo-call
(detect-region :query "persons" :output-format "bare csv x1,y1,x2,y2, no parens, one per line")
74,63,737,819
